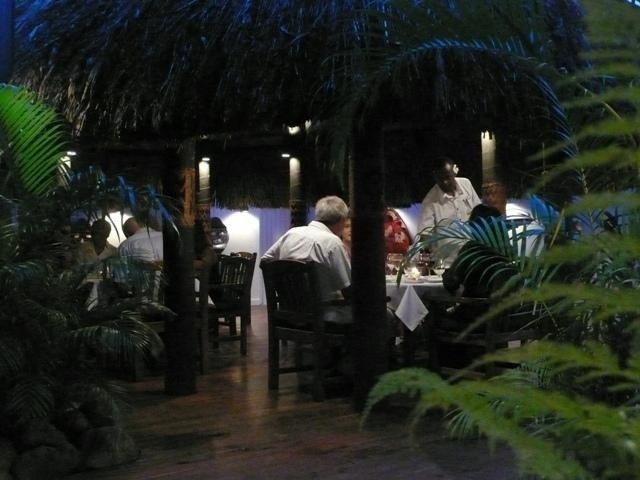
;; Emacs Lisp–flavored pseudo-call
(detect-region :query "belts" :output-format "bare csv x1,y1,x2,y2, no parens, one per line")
327,300,348,306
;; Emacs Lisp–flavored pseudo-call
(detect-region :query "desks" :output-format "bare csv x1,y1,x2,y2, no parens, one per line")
385,267,452,369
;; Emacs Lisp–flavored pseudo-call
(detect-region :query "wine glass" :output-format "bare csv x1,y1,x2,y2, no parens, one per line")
386,250,404,284
418,252,445,278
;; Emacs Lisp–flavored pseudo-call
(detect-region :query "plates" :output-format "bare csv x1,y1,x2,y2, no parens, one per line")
419,275,442,282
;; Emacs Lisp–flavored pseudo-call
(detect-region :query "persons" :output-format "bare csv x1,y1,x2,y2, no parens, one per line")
75,219,119,273
341,208,352,253
262,194,353,326
116,217,163,297
419,156,482,251
441,204,525,296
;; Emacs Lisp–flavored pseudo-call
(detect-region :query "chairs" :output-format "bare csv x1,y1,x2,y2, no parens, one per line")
419,286,538,375
99,249,355,405
